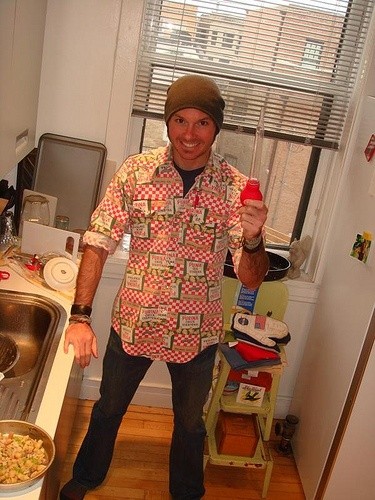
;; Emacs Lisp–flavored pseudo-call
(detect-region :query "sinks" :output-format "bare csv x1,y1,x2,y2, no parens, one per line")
0,289,68,379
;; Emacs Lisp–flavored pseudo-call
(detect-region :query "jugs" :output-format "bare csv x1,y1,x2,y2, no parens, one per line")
21,194,51,230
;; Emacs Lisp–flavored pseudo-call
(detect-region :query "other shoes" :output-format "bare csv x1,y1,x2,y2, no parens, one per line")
60,479,88,500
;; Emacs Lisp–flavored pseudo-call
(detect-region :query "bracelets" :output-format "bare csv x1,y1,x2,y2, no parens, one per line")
71,304,92,317
68,315,92,325
244,234,261,250
241,237,263,253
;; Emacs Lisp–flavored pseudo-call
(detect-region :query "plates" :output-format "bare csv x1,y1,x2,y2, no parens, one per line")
0,419,55,492
43,256,79,292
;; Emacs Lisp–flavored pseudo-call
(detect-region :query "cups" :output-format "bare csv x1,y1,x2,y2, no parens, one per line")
0,211,18,244
54,215,70,230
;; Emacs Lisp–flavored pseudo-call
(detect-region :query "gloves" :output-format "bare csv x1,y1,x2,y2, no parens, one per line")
231,313,290,353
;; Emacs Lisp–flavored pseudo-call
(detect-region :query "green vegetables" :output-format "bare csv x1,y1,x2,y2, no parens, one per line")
0,436,39,483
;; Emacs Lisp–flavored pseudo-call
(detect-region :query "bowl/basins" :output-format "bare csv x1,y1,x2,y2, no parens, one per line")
0,332,20,373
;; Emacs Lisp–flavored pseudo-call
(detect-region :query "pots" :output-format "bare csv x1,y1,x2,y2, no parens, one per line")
223,249,292,281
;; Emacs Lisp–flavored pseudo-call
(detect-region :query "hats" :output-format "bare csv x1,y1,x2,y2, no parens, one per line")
164,75,225,135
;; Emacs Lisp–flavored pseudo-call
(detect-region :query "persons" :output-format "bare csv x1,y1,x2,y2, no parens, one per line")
63,74,269,500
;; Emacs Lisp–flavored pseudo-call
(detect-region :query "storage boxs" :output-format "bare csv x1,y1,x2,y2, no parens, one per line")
213,411,260,458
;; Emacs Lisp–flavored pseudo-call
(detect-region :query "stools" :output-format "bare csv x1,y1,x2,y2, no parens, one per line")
204,277,290,438
203,420,274,498
